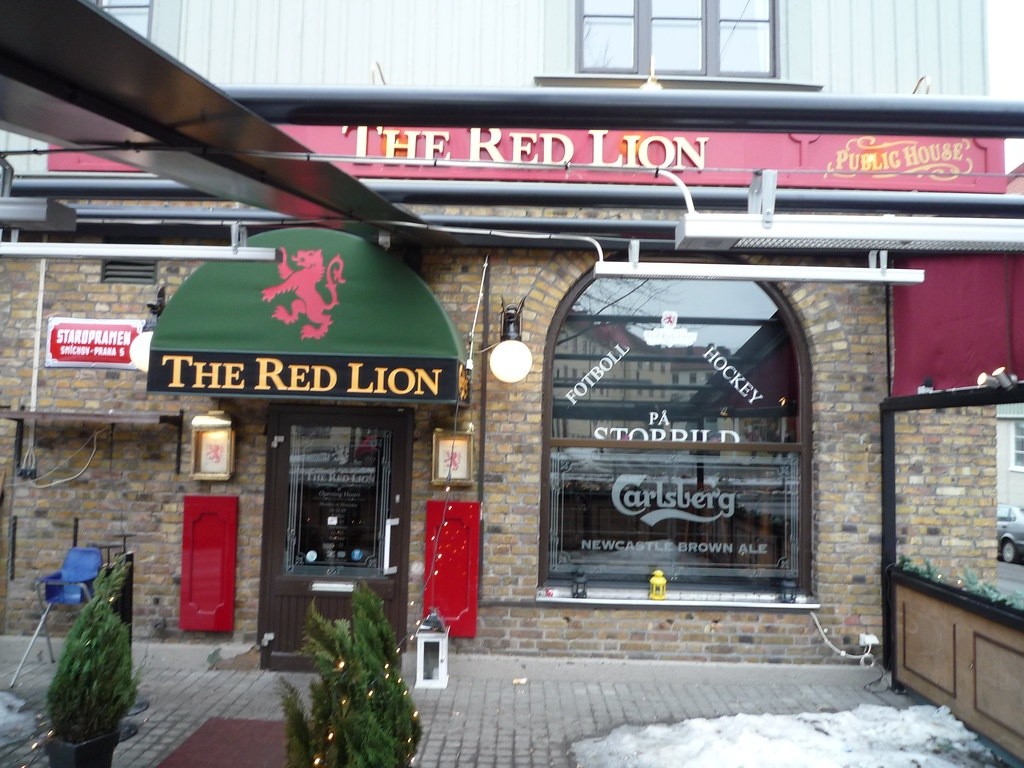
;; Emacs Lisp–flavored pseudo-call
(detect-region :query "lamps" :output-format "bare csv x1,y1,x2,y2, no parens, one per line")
488,303,533,383
647,570,667,598
572,568,590,597
992,367,1018,389
129,285,168,372
976,372,995,389
781,572,799,602
641,53,663,91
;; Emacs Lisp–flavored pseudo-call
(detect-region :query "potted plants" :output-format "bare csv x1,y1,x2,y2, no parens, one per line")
39,559,144,768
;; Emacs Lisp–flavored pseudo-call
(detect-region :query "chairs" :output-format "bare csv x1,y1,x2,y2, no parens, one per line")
8,548,104,689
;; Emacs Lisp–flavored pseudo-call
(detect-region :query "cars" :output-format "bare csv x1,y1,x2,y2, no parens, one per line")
996,503,1024,563
